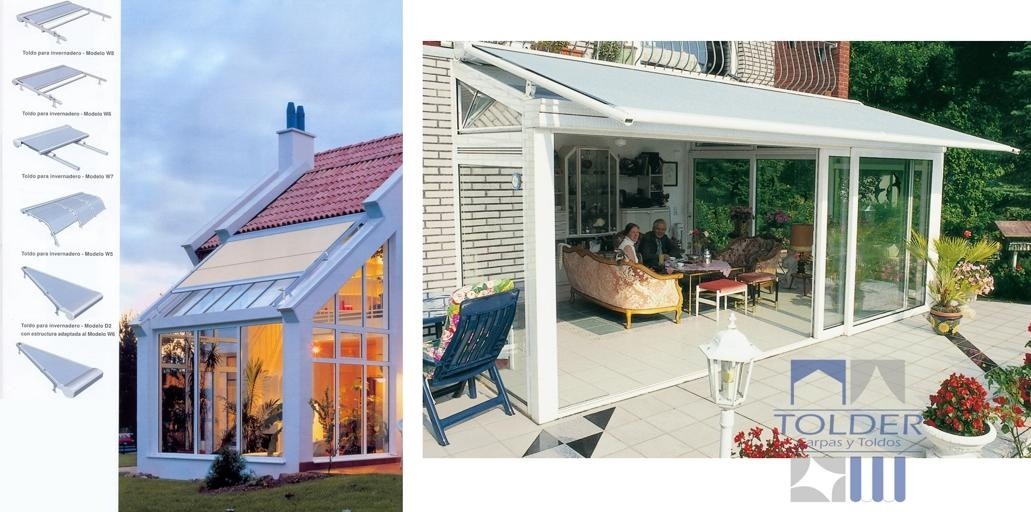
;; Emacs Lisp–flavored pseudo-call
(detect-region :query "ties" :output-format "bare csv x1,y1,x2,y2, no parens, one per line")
657,239,661,257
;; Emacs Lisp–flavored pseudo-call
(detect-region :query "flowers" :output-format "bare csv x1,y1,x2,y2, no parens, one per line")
730,208,756,224
688,229,709,241
921,372,999,437
953,261,996,296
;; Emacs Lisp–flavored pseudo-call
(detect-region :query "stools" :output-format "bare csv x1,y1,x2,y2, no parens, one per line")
696,272,778,322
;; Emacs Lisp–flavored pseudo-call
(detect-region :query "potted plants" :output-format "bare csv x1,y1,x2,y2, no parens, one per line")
902,227,1001,336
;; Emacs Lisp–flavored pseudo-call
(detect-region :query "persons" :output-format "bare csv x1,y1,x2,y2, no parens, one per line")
640,218,682,274
617,223,656,273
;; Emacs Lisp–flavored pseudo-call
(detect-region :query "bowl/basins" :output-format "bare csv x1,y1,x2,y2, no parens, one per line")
686,253,700,262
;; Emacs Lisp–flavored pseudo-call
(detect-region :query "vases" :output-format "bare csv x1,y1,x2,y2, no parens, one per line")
736,223,748,231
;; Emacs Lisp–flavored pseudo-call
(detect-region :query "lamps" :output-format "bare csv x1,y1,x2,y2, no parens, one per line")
789,223,814,273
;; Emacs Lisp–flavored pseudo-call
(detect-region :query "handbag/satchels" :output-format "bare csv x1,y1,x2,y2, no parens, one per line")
614,248,628,260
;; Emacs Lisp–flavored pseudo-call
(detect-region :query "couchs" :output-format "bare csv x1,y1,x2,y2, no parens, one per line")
710,233,783,294
562,244,685,329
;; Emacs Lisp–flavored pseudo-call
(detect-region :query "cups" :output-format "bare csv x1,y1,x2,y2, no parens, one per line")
668,257,685,269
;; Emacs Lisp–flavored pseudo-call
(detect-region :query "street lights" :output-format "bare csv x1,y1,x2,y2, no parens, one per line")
699,311,765,458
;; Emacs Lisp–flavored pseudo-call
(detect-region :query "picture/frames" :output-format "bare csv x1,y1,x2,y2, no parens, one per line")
663,162,679,187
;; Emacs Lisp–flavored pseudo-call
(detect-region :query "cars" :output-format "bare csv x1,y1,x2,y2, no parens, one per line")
120,432,135,446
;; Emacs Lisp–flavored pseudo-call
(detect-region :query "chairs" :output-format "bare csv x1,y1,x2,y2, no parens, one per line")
424,287,521,445
